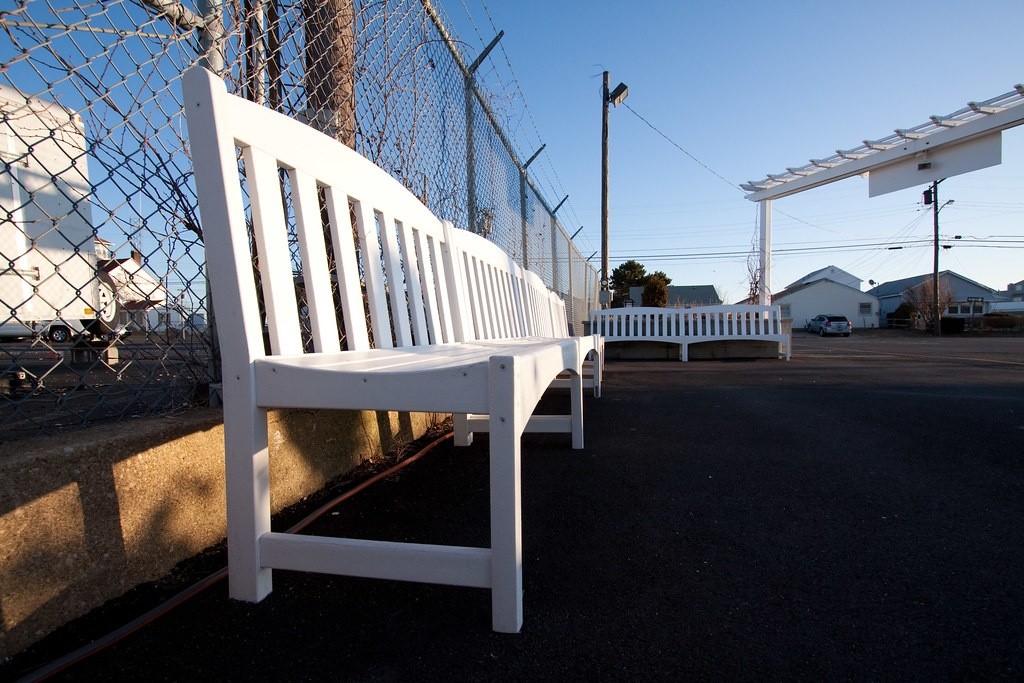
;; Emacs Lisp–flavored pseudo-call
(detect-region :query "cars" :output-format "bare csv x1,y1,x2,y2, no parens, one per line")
809,313,853,337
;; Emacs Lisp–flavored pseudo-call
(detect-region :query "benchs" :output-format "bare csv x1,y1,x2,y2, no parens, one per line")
181,64,584,636
590,306,686,362
520,265,605,385
445,220,602,442
681,304,790,362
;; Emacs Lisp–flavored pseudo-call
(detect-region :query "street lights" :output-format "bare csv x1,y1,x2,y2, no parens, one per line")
600,67,631,310
932,199,956,337
